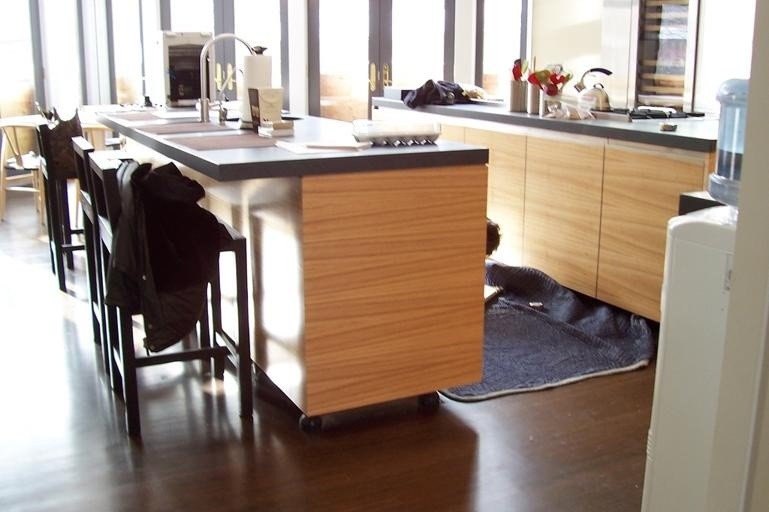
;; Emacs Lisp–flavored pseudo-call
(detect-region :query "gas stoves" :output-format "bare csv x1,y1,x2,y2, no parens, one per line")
587,107,704,121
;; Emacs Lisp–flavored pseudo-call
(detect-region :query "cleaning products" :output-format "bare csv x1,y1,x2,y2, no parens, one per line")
259,88,281,126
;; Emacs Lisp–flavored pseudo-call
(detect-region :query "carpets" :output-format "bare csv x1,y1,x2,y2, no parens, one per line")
434,257,659,403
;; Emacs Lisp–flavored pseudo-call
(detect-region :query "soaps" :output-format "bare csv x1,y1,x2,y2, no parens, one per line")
258,119,294,138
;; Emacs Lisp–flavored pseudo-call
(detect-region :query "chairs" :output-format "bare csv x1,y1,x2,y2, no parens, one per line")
1,96,112,234
35,109,98,293
69,134,212,377
88,150,255,442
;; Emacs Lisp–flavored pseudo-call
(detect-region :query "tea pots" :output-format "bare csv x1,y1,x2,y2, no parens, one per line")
573,67,613,111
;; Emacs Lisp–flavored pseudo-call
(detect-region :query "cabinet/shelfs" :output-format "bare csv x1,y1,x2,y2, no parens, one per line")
521,130,719,330
435,123,527,270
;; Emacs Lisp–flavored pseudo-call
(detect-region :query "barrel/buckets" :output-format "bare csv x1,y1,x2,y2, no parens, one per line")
706,78,751,210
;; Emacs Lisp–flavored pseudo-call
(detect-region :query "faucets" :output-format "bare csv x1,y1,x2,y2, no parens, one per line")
199,34,256,122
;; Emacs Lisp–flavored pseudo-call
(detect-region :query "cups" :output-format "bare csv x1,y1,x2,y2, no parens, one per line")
505,80,527,114
525,81,541,114
538,89,562,118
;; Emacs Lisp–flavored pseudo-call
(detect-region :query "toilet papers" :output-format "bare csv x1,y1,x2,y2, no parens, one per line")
241,54,273,125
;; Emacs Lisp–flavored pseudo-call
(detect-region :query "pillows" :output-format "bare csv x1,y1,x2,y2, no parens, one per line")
1,86,44,157
